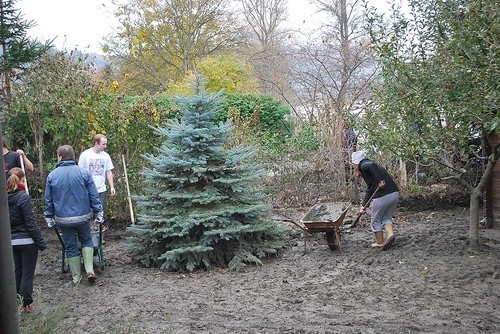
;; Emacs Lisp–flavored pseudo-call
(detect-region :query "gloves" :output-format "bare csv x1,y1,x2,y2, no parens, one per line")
93,211,104,224
45,218,56,228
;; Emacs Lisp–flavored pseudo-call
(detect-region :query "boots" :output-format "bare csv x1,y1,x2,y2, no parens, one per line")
68,256,83,287
82,247,98,282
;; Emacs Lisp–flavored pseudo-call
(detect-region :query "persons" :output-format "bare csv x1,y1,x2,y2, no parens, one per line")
42,145,104,285
5,167,47,313
341,119,360,185
0,138,34,194
77,134,116,244
351,151,400,251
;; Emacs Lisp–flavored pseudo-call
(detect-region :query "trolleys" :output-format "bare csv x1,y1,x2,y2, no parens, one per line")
282,202,352,256
54,223,105,273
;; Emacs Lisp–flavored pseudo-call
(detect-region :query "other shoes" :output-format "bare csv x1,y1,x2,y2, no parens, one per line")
371,243,384,248
383,235,395,250
23,305,33,313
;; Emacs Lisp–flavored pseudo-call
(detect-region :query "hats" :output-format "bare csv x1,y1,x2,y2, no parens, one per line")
351,150,366,164
